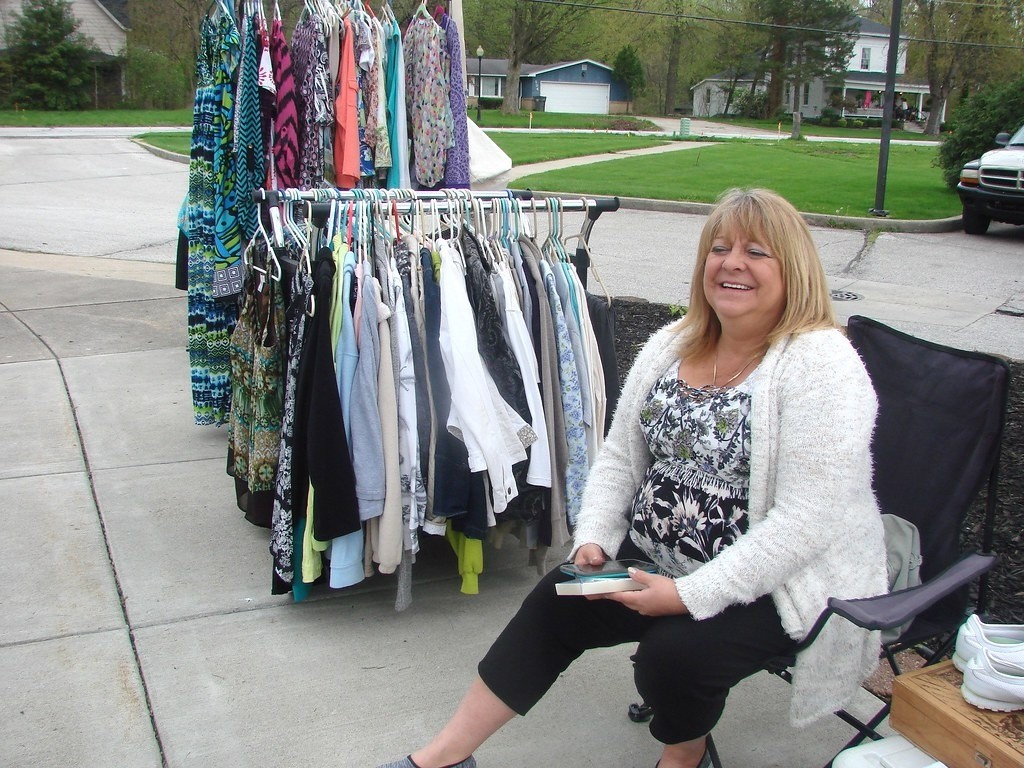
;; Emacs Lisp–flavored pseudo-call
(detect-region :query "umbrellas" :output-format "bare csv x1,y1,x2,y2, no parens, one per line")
864,90,871,108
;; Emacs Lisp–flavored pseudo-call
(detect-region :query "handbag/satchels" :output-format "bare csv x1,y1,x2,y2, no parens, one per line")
880,513,921,647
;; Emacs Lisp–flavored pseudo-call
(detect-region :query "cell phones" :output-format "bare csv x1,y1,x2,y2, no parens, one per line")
560,557,659,581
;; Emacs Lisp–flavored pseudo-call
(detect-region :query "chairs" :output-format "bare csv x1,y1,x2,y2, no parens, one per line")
630,314,1010,768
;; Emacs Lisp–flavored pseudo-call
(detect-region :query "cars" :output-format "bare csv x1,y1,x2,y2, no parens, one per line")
955,123,1024,235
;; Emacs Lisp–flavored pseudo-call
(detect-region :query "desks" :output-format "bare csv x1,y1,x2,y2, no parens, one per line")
888,658,1024,768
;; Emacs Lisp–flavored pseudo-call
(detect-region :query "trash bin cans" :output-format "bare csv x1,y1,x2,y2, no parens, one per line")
679,118,690,136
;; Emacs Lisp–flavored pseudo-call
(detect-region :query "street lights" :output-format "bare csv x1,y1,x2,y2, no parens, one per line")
476,44,485,121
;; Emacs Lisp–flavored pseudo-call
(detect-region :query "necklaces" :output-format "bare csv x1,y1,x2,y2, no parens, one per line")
713,353,762,387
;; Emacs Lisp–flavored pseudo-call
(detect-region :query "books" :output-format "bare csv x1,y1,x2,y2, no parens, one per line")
555,577,646,596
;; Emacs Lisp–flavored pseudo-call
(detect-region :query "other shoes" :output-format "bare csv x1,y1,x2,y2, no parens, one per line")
952,614,1024,675
374,753,476,768
961,646,1024,713
655,745,711,768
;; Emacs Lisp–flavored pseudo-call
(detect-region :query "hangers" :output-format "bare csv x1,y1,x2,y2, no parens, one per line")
198,0,446,37
243,188,612,318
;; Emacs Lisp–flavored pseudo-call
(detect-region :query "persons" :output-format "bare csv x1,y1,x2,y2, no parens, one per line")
896,97,909,121
382,190,887,768
